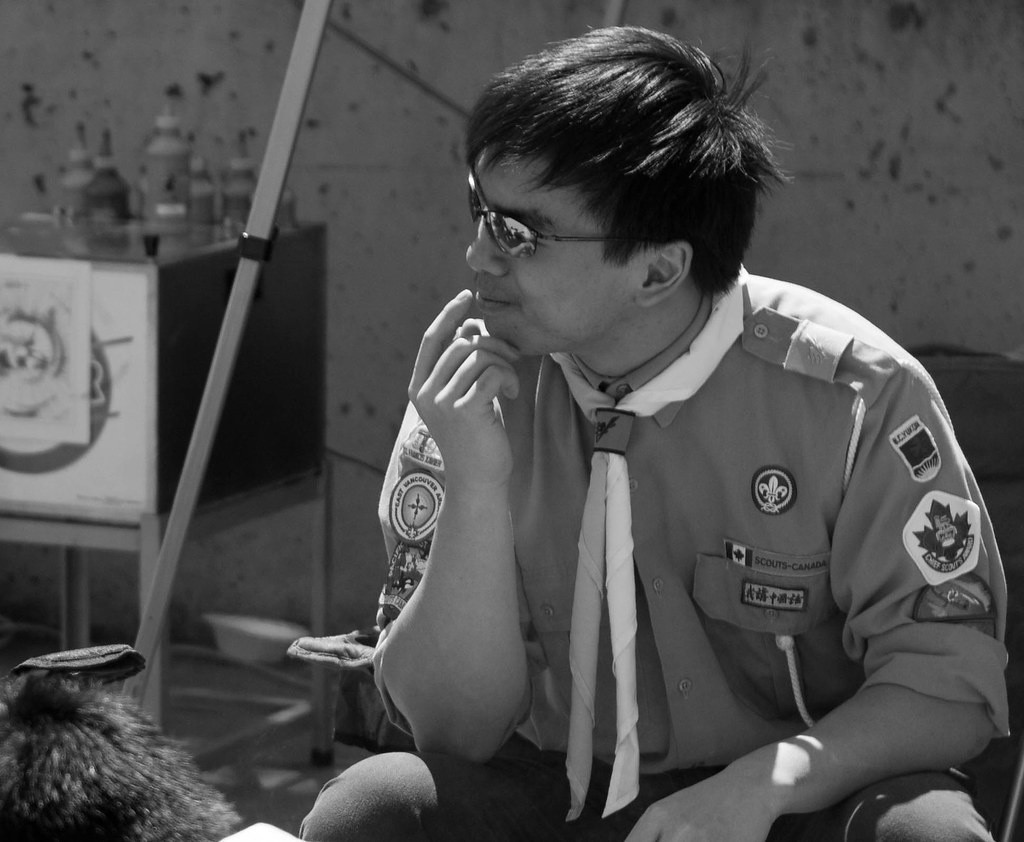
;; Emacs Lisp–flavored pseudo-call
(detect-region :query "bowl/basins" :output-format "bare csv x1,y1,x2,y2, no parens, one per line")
201,611,307,664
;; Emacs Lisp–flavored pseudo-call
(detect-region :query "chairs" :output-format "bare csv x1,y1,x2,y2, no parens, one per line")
913,355,1024,842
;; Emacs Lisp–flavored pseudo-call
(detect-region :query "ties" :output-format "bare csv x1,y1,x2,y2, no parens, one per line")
548,265,752,822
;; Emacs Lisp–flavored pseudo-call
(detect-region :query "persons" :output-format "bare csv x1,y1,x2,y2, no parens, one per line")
295,25,1012,842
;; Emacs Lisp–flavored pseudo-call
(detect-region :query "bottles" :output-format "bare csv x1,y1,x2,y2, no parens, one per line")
83,128,131,221
143,102,192,223
61,119,96,217
275,185,299,228
219,138,258,226
186,154,215,224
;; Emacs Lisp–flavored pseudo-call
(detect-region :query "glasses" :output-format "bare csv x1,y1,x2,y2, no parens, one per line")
465,169,664,260
